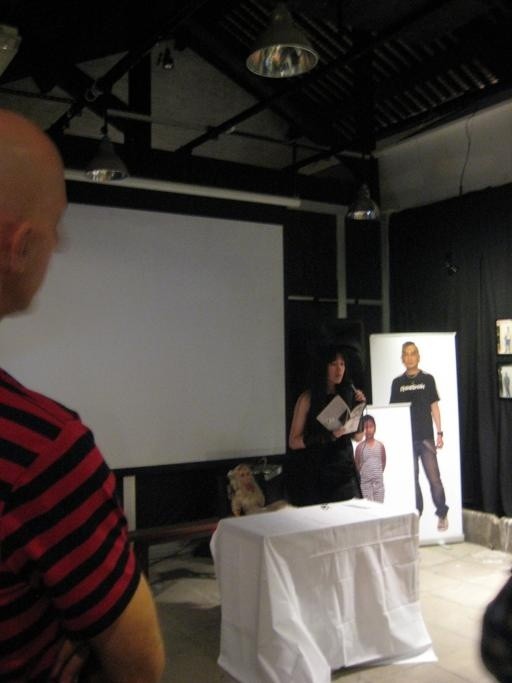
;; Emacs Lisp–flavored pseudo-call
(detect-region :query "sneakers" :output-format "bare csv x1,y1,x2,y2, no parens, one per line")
436,515,450,532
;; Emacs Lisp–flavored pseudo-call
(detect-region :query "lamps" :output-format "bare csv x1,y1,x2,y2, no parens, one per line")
162,46,175,71
346,182,384,222
244,2,323,78
85,109,129,182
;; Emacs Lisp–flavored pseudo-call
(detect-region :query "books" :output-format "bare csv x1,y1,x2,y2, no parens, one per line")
316,393,366,436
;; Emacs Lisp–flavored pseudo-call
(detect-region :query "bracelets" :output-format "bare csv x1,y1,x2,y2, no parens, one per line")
438,431,443,435
303,432,321,448
324,431,334,445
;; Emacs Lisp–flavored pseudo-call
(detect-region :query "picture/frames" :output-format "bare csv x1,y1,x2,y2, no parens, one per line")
496,319,512,399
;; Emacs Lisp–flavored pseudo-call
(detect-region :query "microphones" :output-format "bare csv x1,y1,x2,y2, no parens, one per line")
343,376,366,404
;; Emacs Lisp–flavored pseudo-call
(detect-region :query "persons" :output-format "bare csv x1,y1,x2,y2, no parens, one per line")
355,414,387,502
288,346,367,506
388,340,449,530
2,106,168,683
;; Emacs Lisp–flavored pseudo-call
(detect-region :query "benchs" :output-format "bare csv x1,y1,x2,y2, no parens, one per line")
118,515,248,582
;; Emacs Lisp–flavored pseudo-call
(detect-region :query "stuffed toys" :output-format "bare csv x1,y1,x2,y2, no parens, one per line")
226,461,293,515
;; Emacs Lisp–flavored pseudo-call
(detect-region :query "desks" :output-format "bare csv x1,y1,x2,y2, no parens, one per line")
210,497,437,683
